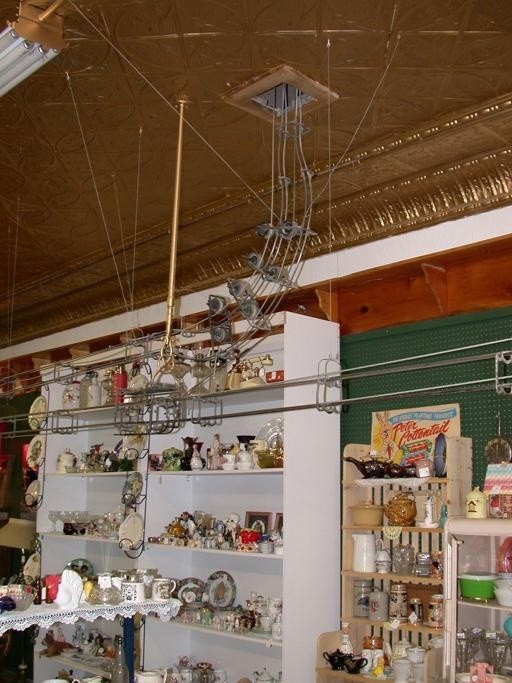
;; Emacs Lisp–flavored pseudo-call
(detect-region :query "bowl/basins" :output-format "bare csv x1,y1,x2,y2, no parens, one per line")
0,584,38,612
458,571,498,600
350,503,384,526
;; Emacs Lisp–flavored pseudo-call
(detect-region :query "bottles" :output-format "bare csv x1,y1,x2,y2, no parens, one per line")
190,434,223,471
62,364,128,410
33,576,42,604
340,624,354,655
352,580,443,628
112,637,213,682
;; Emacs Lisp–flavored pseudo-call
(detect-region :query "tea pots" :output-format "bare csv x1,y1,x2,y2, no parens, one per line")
385,492,417,526
351,533,383,573
385,637,418,671
323,649,346,671
253,668,277,683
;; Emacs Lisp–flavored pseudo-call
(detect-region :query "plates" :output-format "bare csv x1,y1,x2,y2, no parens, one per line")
173,571,236,611
455,673,511,683
22,552,40,584
434,433,448,477
25,395,47,506
119,375,148,551
498,536,511,574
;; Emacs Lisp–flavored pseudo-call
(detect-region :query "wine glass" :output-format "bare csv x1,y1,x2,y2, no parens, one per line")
48,511,91,537
456,629,509,673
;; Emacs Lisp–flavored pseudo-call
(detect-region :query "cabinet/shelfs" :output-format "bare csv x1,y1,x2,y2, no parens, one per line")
315,403,474,683
443,476,512,683
36,309,341,683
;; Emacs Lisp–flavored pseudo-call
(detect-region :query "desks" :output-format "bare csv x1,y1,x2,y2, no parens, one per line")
0,574,183,683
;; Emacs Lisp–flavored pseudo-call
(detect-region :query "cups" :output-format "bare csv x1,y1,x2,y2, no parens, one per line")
214,670,226,682
151,578,176,603
345,656,368,674
272,623,282,641
392,646,427,682
